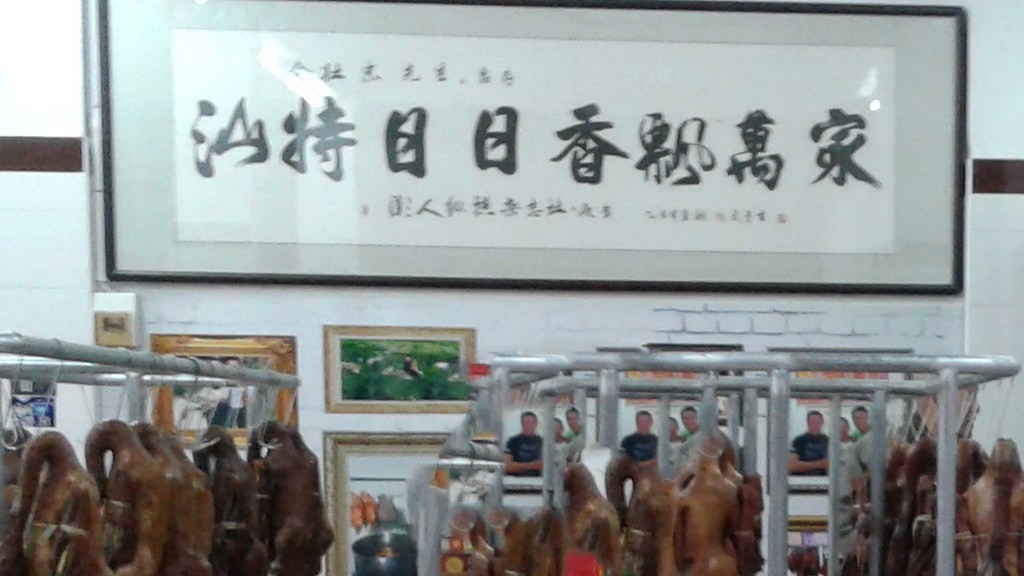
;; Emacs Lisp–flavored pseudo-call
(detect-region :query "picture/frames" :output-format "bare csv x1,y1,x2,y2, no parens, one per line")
98,0,968,296
323,431,456,576
149,332,297,447
322,324,479,413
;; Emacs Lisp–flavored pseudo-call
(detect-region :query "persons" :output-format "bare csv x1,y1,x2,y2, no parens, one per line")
852,407,872,439
839,418,858,444
173,357,250,428
668,406,700,463
620,409,658,462
504,410,543,477
787,410,829,476
554,407,580,447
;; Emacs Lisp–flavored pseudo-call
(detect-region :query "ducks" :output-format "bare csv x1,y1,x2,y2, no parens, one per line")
840,434,1024,575
466,431,764,575
0,421,334,576
350,491,377,531
